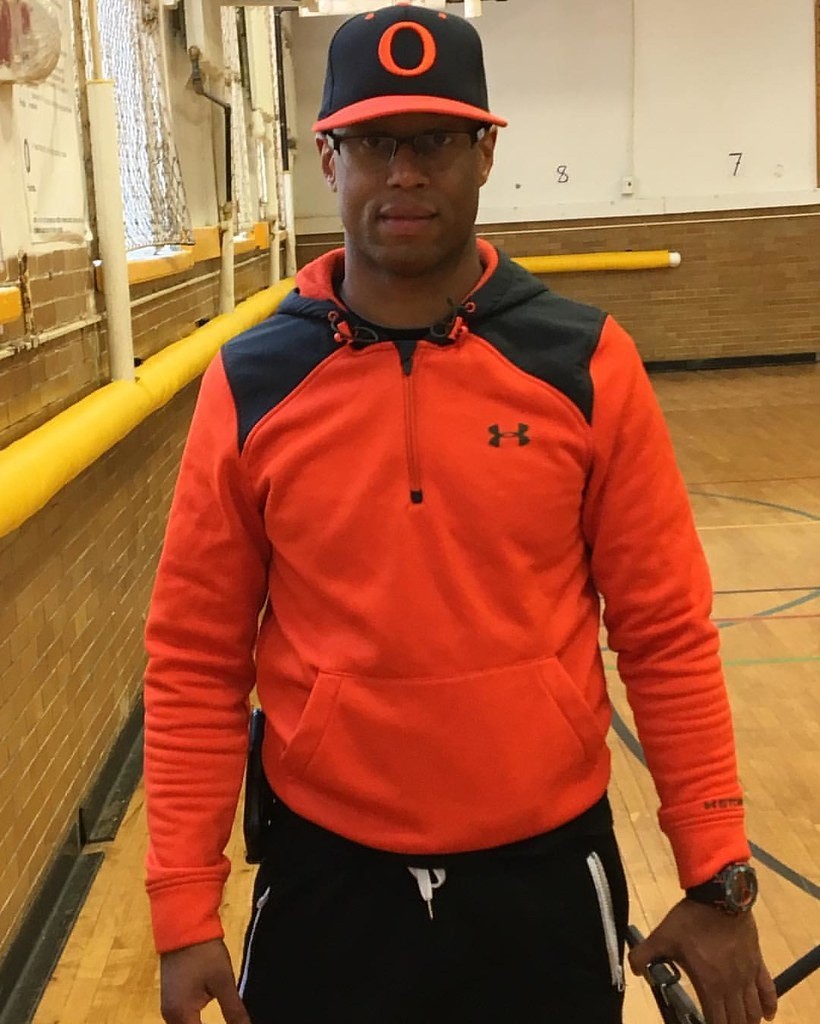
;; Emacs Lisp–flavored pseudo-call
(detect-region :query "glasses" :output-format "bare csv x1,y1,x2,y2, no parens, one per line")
327,123,492,170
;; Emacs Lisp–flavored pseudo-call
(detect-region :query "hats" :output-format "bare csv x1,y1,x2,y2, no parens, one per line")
311,2,508,132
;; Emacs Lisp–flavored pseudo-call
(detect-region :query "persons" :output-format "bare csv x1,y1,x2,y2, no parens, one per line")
143,4,784,1024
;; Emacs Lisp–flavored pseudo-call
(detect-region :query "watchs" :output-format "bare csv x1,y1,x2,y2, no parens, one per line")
687,861,760,917
